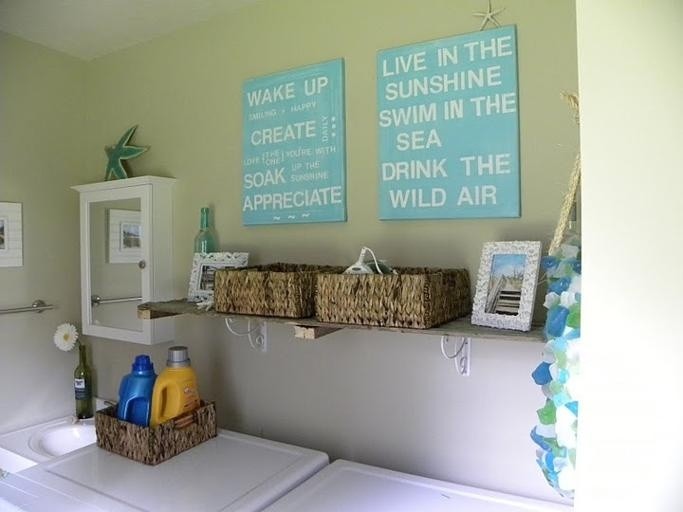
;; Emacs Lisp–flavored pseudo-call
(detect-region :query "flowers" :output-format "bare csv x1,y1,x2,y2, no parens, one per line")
54,324,81,352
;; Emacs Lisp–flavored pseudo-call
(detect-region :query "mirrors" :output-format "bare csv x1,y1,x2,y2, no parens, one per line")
88,198,142,332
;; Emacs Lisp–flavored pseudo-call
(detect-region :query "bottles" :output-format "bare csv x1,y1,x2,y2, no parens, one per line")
73,344,93,420
195,207,216,254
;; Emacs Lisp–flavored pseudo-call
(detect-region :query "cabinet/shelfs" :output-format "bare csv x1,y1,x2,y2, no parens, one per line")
68,174,176,346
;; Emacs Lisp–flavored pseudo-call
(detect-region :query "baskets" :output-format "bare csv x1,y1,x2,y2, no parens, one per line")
94,396,218,467
214,261,343,318
313,264,472,330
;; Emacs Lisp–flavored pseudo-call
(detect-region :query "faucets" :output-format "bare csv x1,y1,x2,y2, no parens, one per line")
103,399,117,408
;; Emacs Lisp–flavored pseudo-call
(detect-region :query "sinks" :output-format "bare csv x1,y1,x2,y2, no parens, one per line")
1,413,104,472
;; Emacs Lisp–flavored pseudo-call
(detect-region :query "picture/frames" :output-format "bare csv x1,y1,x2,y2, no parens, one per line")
0,202,23,267
108,208,142,264
472,241,541,333
188,251,248,304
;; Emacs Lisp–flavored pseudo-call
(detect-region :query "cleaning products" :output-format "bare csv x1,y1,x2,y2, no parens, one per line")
149,343,202,426
115,352,160,426
